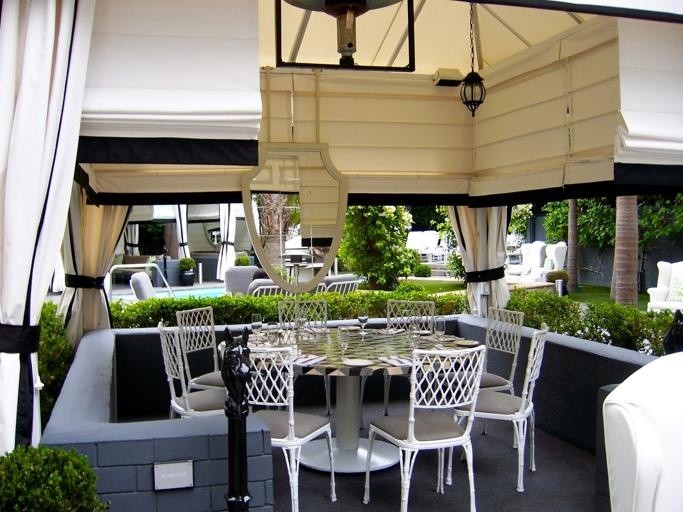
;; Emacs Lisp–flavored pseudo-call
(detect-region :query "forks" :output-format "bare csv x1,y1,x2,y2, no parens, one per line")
385,352,405,364
293,355,310,363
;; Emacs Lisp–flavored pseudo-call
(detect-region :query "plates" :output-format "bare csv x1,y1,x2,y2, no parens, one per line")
454,340,480,346
410,330,431,335
339,325,360,332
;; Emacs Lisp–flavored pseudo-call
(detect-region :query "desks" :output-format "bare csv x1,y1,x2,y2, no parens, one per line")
216,326,477,475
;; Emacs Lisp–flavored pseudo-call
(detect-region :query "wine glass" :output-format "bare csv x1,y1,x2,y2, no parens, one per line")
251,313,262,344
434,317,446,347
357,307,369,335
336,326,349,362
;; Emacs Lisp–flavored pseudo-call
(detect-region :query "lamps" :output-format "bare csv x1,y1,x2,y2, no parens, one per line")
459,2,487,117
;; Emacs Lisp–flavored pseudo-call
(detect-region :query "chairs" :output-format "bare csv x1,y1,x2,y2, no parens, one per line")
362,345,486,511
223,251,360,294
436,321,549,492
129,272,155,302
505,240,568,298
406,229,453,263
221,345,339,512
175,305,271,415
356,299,436,430
444,306,525,449
646,260,683,313
601,349,683,512
276,300,331,415
158,321,253,419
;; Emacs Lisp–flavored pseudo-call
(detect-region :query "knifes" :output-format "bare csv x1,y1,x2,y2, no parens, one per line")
301,354,320,365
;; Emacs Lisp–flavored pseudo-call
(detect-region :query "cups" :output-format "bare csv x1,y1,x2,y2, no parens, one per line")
408,318,421,339
267,330,278,345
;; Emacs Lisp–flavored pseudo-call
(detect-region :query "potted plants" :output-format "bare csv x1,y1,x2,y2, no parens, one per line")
178,256,196,286
